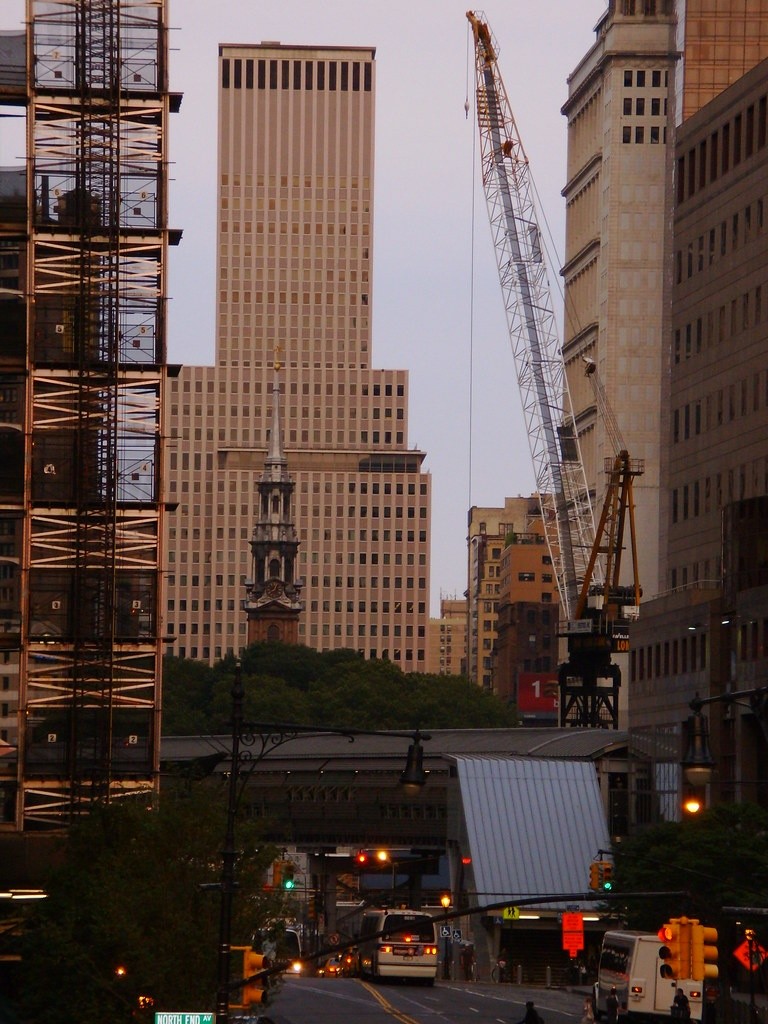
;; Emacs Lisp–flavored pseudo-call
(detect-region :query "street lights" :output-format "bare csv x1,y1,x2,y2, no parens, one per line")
746,923,761,1024
440,894,452,981
217,654,433,1024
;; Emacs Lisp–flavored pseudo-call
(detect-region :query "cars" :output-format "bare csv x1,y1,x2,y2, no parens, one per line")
315,944,359,979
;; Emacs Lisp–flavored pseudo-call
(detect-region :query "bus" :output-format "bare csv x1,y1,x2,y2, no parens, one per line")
357,907,437,985
253,924,302,982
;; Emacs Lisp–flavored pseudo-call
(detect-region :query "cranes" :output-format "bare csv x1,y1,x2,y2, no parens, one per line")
464,8,646,654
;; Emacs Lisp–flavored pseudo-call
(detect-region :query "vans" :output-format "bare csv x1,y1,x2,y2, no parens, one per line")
593,931,703,1024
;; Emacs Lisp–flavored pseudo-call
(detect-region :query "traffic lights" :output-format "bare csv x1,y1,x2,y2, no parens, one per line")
691,919,719,983
588,860,600,892
681,800,703,818
281,862,296,892
659,922,691,980
602,861,614,893
349,846,394,865
241,946,268,1009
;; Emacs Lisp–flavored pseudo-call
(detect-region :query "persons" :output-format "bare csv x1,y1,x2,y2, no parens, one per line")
583,999,595,1024
606,988,620,1024
459,949,474,981
672,988,691,1024
515,1001,546,1024
